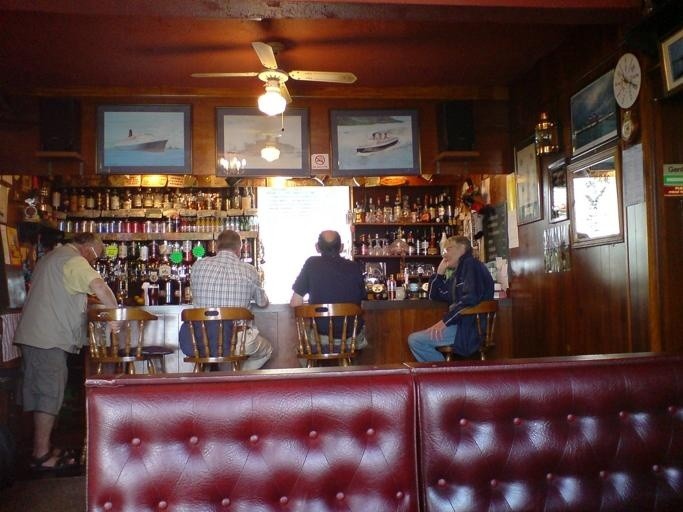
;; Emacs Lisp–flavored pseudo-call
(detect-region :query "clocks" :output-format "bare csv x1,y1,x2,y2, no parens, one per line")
611,51,643,110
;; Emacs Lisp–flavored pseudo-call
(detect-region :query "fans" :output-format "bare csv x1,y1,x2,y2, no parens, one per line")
189,40,358,104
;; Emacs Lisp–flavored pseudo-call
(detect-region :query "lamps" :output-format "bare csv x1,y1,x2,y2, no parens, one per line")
255,80,288,119
259,141,282,163
214,154,247,177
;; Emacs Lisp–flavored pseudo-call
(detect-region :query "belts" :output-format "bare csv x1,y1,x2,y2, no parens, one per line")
234,326,250,332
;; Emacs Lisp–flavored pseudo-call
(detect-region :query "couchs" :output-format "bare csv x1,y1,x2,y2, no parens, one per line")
85,351,683,512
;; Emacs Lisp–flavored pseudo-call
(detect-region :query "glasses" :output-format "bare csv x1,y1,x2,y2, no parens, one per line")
442,245,460,255
90,246,99,262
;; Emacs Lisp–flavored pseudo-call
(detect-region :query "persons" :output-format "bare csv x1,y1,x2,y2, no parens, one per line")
290,230,365,367
12,232,117,470
190,230,274,371
407,235,494,362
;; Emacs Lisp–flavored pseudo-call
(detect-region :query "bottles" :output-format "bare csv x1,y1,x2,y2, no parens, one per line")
121,187,181,209
182,216,258,231
242,240,249,260
182,279,192,304
354,192,455,223
92,260,191,289
104,240,218,260
51,187,121,210
359,225,454,256
387,274,396,301
221,188,254,209
163,278,181,303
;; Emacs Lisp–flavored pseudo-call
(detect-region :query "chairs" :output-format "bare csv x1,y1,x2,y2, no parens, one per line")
436,300,501,362
293,301,364,369
86,306,163,376
179,306,255,374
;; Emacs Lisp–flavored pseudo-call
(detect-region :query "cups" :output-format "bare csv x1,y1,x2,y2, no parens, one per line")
396,288,406,301
149,284,158,306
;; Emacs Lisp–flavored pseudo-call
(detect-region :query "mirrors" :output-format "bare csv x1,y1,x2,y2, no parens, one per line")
565,144,626,250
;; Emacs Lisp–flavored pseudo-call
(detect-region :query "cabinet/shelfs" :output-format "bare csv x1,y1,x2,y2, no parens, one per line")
349,174,469,301
18,172,261,315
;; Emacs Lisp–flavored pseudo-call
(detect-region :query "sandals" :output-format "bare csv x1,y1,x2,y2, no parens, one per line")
30,452,75,478
37,444,78,461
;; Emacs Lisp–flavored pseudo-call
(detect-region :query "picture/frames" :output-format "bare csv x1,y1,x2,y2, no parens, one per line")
213,105,312,178
546,155,570,225
94,103,193,176
512,134,542,228
568,66,619,163
661,29,683,92
327,107,422,178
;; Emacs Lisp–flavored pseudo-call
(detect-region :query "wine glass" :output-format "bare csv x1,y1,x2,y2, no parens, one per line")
115,279,129,308
